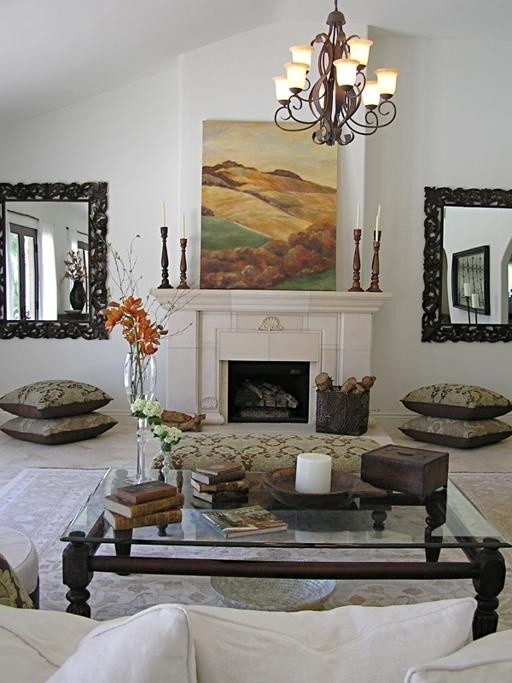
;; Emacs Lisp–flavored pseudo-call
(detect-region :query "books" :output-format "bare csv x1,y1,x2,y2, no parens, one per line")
188,462,251,509
102,479,186,530
201,504,289,538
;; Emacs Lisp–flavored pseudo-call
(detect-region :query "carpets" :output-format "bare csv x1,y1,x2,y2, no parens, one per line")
0,467,511,632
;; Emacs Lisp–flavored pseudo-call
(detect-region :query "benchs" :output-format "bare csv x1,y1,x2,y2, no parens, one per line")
149,433,380,473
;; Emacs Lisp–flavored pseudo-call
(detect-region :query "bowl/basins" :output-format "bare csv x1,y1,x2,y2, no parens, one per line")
263,466,362,508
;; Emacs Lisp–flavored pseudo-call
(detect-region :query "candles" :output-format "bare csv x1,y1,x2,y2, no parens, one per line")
375,216,379,241
356,204,360,228
472,294,479,308
182,211,185,238
464,283,471,296
163,203,166,226
378,204,381,224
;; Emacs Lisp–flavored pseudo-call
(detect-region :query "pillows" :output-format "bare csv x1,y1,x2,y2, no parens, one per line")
399,382,512,421
0,412,119,446
398,415,512,450
0,379,114,420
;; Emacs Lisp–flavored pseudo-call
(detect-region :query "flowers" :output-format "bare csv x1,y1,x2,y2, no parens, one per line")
60,250,88,283
128,399,182,452
74,217,199,399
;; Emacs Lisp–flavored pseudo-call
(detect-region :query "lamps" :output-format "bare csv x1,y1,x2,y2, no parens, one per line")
271,0,398,147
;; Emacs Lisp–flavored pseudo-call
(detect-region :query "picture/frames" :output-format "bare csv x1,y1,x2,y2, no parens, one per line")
451,245,490,317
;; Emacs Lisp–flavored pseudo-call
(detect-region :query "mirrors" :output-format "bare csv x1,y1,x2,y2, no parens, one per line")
0,180,110,341
421,186,511,344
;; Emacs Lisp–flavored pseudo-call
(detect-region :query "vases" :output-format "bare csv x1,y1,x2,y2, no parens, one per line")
123,352,157,485
70,281,86,311
149,449,176,488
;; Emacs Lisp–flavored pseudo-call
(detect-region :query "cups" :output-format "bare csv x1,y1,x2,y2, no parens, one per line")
295,454,331,493
